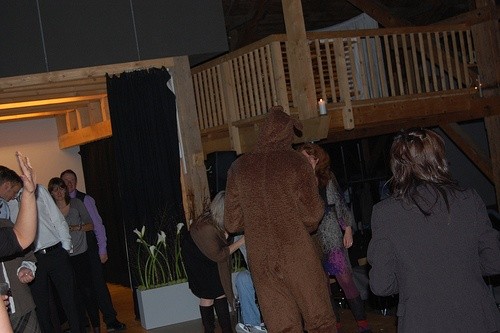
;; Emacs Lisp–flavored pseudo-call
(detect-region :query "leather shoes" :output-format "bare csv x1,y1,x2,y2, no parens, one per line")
107,320,126,332
94,327,100,333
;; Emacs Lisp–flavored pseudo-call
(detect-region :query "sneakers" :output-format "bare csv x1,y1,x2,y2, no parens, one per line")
235,323,251,333
250,323,268,333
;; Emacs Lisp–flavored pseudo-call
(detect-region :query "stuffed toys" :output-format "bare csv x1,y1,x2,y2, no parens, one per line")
223,105,338,333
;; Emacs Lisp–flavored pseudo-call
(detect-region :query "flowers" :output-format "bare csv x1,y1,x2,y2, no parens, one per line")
133,223,188,288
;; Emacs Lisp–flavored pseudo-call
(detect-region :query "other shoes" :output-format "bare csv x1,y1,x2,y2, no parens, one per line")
337,320,343,333
360,326,372,333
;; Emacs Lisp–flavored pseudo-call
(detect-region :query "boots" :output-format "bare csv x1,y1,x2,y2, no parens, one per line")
199,304,216,333
214,297,233,333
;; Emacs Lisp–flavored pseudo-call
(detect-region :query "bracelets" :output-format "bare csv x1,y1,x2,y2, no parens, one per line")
78,224,81,231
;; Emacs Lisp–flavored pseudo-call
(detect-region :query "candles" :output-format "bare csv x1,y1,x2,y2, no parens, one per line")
318,99,327,115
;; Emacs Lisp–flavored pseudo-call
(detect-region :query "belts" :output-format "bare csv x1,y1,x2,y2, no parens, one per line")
34,242,62,256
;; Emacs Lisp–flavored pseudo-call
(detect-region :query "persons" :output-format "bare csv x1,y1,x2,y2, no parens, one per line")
0,166,126,333
180,142,373,333
367,126,500,333
